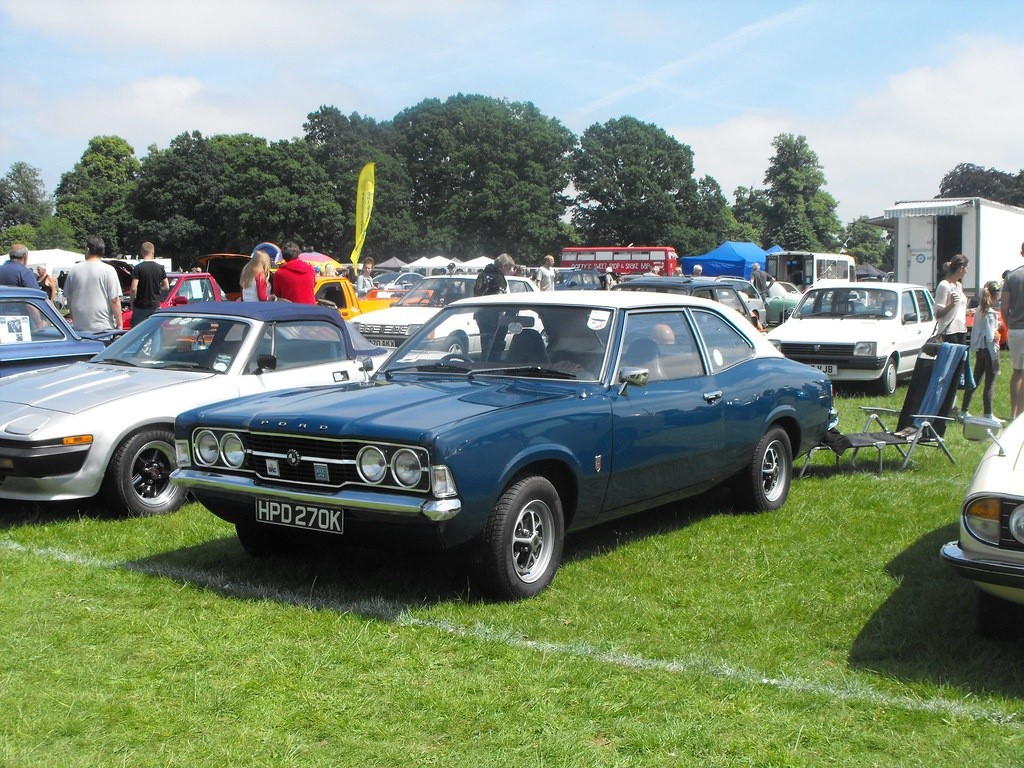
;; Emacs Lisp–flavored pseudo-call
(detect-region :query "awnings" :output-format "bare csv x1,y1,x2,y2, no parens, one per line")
883,202,975,220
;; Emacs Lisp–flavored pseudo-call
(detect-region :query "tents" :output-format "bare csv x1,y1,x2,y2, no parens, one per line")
843,262,887,282
408,256,468,277
681,240,797,280
462,255,495,269
374,255,408,269
401,256,429,273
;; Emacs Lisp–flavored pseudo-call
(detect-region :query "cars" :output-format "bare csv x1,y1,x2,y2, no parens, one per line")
965,306,1008,350
939,409,1024,611
760,280,942,397
0,298,387,521
0,252,808,388
165,288,836,601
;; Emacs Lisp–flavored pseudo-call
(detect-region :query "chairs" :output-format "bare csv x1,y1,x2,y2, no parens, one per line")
504,328,553,367
797,343,970,479
614,338,669,385
443,286,462,305
228,326,269,373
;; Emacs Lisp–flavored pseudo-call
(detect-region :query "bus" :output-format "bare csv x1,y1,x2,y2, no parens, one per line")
559,245,680,276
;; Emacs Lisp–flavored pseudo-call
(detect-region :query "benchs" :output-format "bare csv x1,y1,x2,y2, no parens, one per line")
547,350,737,384
220,340,338,363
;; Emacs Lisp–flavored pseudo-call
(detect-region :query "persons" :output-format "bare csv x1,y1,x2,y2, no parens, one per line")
240,250,271,302
128,241,169,359
749,263,775,327
693,265,704,276
536,255,559,292
643,266,657,277
36,264,56,304
440,263,464,294
1001,242,1024,420
605,266,621,291
473,253,516,362
957,280,1008,424
62,235,123,346
272,244,315,305
312,257,377,297
0,244,50,329
934,255,970,417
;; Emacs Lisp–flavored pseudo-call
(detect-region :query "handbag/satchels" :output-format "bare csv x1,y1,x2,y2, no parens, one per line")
922,334,944,358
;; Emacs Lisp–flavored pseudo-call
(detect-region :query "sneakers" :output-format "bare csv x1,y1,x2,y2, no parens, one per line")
959,411,972,419
985,413,1006,423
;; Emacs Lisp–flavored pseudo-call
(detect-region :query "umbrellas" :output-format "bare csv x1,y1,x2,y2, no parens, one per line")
298,252,345,272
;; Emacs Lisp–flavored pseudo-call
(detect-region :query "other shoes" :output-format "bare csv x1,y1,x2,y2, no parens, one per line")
951,407,962,415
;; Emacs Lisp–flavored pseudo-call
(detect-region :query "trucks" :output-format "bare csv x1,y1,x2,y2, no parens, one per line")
765,250,858,295
882,195,1024,307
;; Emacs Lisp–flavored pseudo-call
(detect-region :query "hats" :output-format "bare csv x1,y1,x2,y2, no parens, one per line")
447,263,455,269
